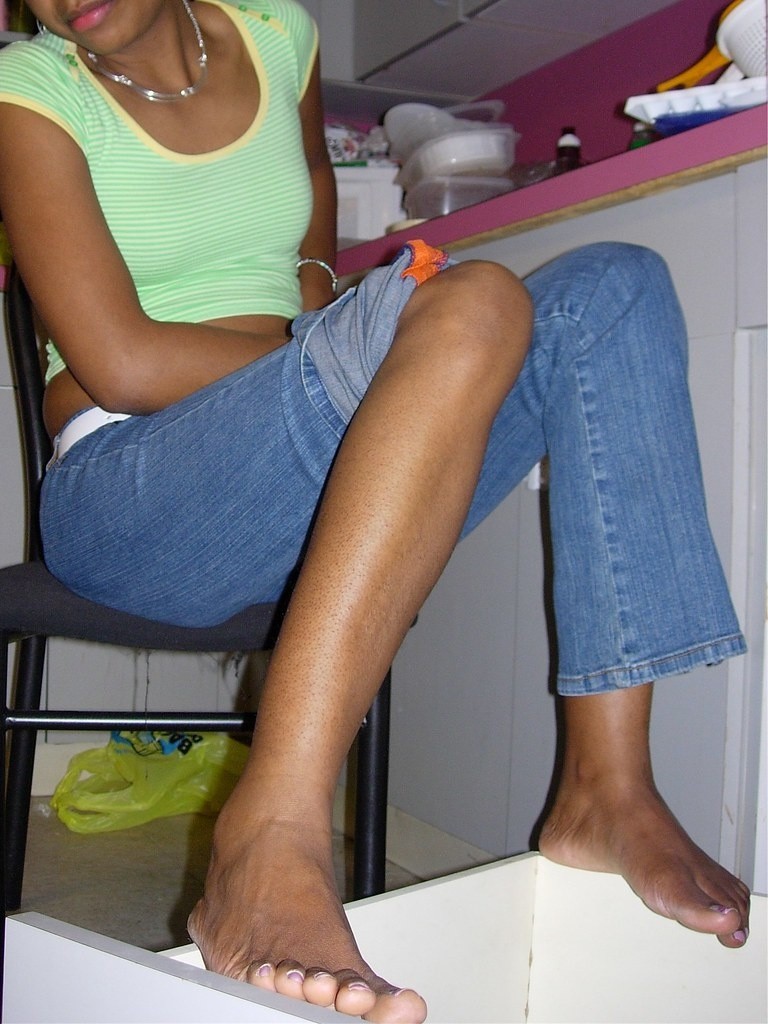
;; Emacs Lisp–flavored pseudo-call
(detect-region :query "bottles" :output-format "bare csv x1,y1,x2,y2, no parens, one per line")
557,126,581,172
628,122,655,150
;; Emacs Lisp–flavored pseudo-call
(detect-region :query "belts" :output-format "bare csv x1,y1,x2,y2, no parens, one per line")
57,405,131,461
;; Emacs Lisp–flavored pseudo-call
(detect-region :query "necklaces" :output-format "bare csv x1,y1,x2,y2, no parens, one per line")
87,0,208,103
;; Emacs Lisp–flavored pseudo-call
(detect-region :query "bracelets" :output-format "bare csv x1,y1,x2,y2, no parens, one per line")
297,257,337,291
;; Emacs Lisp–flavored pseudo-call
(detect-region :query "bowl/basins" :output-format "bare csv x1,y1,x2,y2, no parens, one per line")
394,125,521,221
383,103,454,143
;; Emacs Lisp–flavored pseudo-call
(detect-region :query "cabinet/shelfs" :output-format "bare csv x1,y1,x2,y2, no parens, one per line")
0,102,767,880
353,0,675,96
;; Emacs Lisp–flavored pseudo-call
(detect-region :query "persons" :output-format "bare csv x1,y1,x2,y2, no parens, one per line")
0,0,752,1024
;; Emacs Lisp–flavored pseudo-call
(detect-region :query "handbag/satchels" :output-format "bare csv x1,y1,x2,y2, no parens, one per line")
48,728,255,834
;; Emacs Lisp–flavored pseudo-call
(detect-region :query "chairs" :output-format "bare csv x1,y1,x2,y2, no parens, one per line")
1,256,393,925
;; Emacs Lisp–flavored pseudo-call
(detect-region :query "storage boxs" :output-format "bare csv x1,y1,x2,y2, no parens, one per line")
0,856,768,1024
